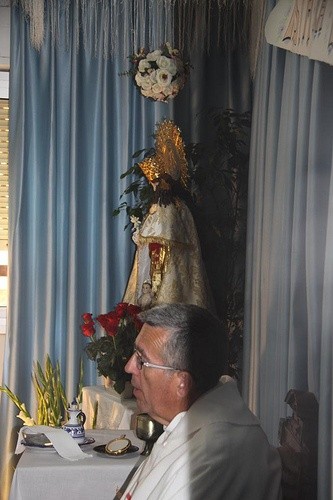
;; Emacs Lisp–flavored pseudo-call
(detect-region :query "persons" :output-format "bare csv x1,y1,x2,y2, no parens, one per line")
117,302,284,500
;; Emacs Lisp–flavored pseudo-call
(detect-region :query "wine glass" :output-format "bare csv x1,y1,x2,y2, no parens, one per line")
135,412,163,456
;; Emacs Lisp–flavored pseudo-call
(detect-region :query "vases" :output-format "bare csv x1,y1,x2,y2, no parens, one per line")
104,378,136,400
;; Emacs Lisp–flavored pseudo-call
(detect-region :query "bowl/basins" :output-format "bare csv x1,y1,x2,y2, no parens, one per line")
21,428,53,447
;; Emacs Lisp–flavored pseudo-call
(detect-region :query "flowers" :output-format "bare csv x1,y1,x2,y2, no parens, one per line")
129,213,141,232
118,40,197,102
81,304,146,399
0,353,69,427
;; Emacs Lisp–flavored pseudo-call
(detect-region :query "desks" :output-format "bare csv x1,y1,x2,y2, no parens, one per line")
10,429,145,500
82,386,142,428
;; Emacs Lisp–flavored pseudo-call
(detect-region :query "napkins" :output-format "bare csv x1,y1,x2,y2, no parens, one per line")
15,424,94,460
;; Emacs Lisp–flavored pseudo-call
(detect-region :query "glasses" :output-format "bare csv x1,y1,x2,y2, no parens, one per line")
132,348,178,371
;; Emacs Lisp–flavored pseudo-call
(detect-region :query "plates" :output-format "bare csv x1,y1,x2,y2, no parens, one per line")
91,444,140,459
20,436,95,453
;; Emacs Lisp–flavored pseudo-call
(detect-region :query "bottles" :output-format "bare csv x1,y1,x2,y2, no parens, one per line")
62,399,86,437
